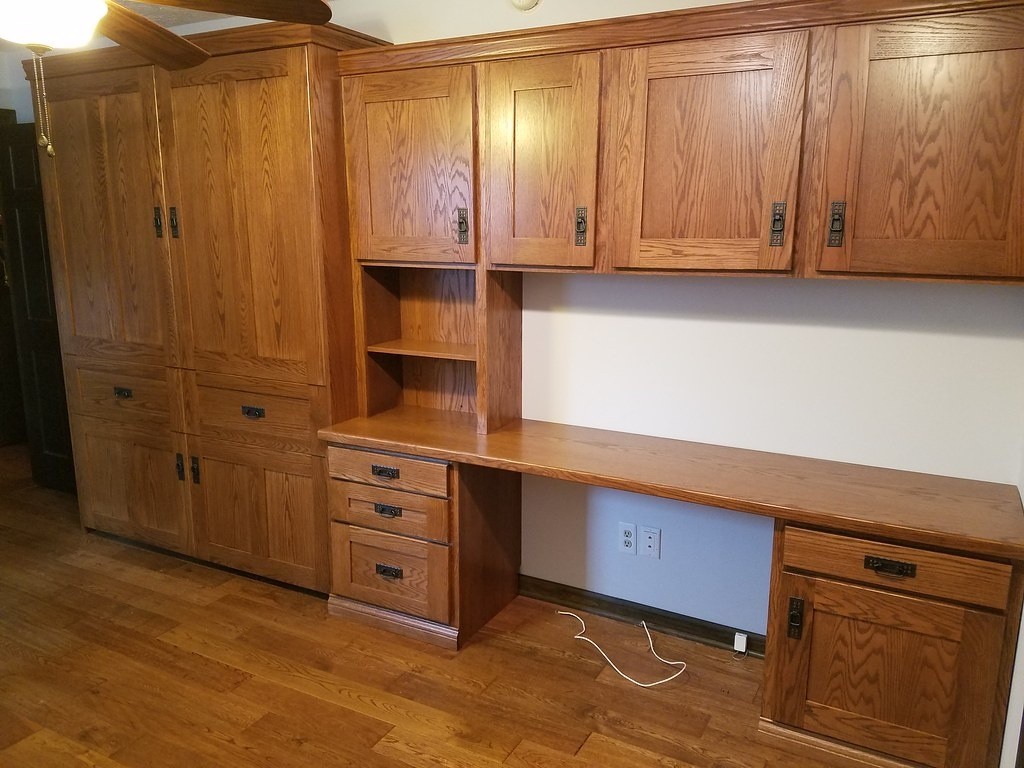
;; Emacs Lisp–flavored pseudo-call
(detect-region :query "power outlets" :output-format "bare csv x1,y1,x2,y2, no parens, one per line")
619,521,637,555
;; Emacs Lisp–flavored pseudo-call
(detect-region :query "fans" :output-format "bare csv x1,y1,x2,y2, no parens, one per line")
0,0,333,72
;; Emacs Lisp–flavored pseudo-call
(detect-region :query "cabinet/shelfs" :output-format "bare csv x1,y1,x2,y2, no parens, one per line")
19,0,1024,596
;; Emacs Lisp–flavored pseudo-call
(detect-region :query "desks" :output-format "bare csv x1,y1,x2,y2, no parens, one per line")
315,408,1024,768
